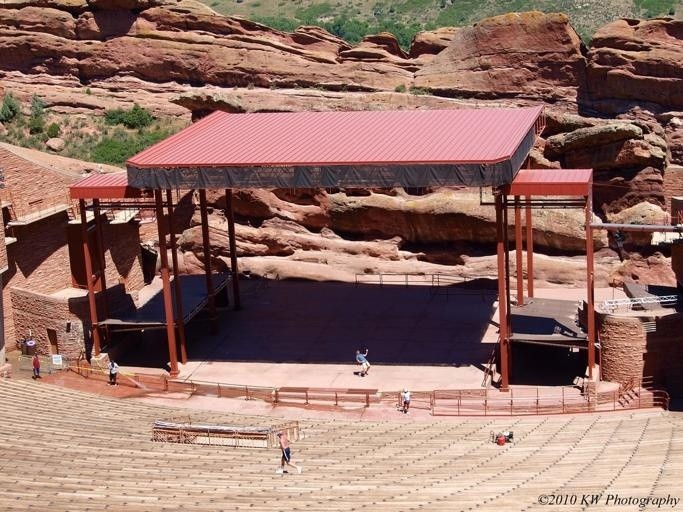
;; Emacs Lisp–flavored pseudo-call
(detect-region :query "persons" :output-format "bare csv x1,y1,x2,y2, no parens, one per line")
401,388,411,414
31,352,41,380
107,360,120,387
495,433,506,445
355,350,371,376
276,431,303,476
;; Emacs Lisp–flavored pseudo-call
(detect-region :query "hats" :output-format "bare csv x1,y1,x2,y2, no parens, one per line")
404,388,408,392
277,431,283,435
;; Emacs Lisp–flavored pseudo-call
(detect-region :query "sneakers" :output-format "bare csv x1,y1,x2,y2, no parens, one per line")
276,469,283,474
297,466,302,474
